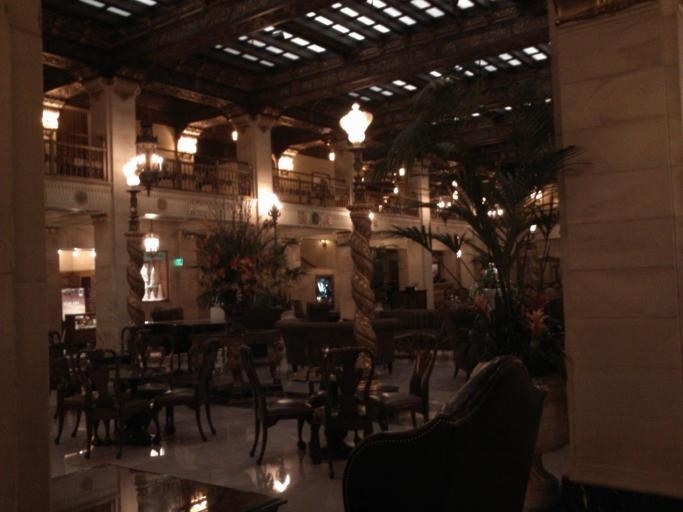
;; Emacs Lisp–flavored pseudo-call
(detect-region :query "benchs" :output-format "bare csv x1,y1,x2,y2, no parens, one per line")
288,188,335,206
158,170,232,193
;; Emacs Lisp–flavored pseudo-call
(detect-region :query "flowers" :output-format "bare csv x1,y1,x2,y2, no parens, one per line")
186,203,298,310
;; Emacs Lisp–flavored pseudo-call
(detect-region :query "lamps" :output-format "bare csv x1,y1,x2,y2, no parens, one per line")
141,219,161,253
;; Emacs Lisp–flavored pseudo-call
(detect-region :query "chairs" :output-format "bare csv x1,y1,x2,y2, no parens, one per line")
48,307,548,512
